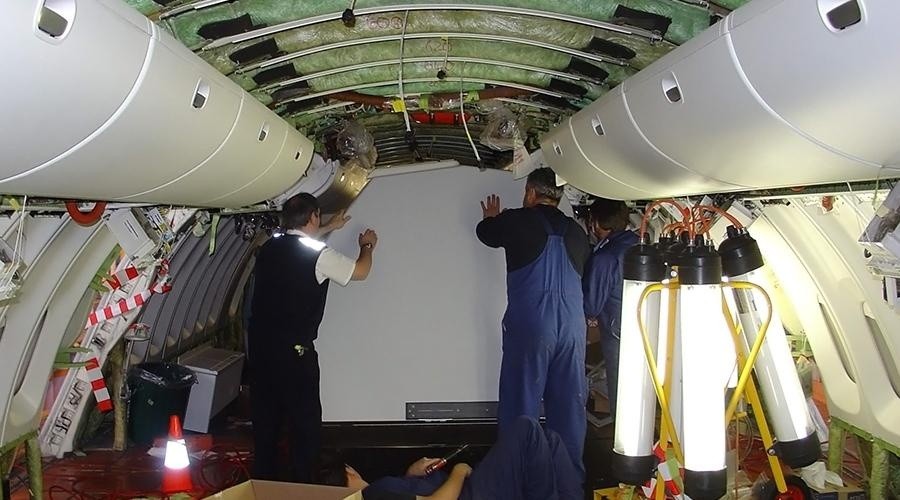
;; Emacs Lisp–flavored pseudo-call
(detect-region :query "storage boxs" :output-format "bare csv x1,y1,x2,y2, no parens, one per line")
201,479,362,500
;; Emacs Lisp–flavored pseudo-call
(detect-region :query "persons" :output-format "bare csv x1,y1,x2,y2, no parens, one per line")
475,167,593,485
293,412,587,500
580,197,641,424
249,191,379,484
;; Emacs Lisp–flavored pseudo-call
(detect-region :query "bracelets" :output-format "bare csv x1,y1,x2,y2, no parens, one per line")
360,242,374,250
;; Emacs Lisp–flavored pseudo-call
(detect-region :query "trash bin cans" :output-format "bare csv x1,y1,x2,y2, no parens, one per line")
127,362,199,446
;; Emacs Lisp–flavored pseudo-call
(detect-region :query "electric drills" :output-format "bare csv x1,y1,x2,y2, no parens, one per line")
425,443,470,476
